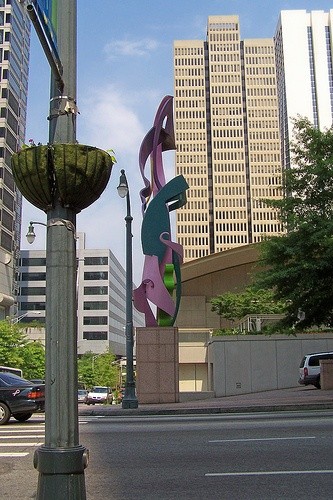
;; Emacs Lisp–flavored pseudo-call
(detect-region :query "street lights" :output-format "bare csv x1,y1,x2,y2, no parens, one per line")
115,168,139,409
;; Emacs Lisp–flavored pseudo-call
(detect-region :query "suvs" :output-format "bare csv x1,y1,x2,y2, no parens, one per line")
298,352,332,389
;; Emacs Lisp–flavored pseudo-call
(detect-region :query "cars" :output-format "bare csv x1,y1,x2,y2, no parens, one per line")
87,386,113,406
30,378,45,385
0,371,45,426
119,388,125,401
77,389,89,405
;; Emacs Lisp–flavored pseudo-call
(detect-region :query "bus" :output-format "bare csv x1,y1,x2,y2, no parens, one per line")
0,366,23,378
